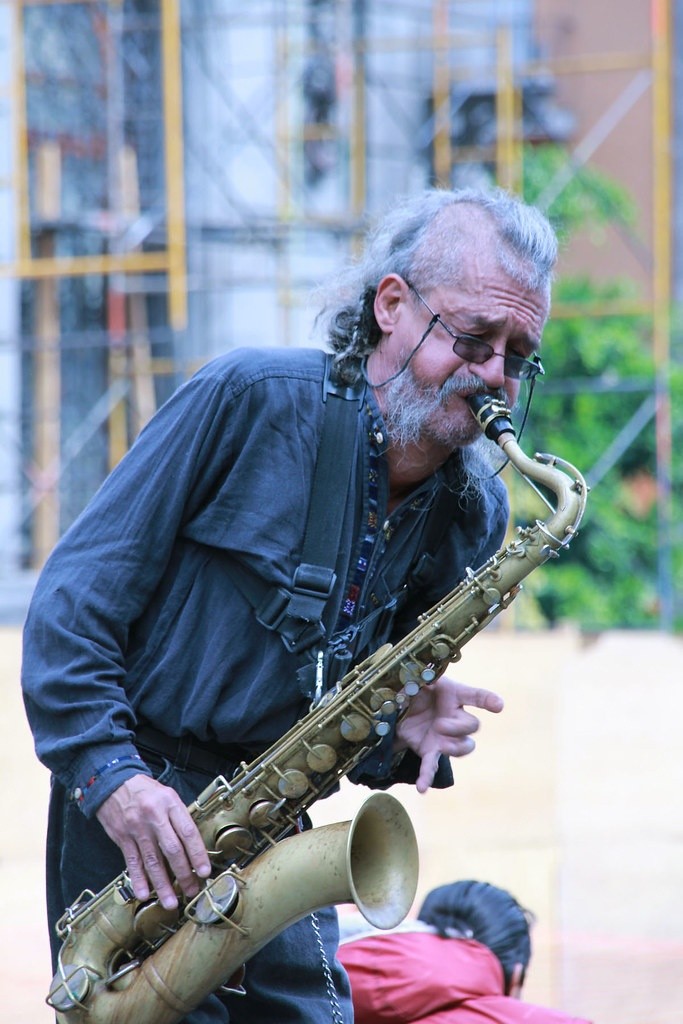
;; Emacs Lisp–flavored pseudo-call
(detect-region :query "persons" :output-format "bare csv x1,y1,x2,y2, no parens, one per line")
336,880,597,1024
21,188,558,1024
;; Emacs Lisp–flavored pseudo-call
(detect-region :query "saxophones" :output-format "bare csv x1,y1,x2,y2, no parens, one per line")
44,396,596,1023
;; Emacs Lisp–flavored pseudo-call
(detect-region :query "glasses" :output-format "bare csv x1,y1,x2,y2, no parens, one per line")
407,279,546,382
